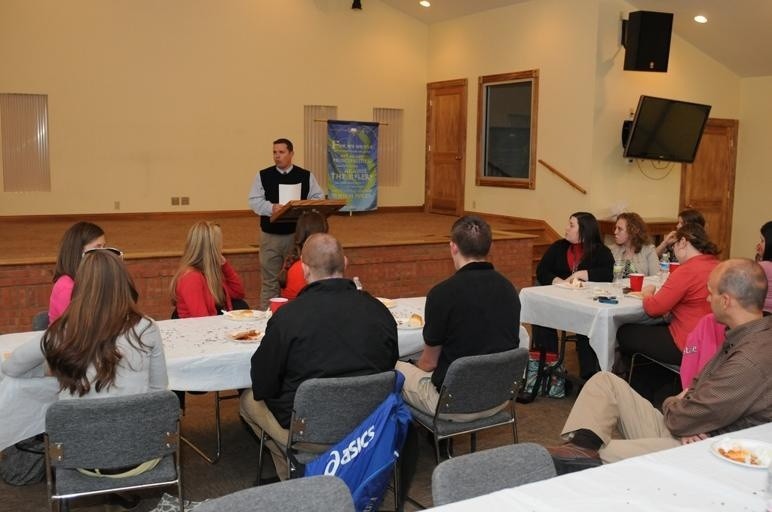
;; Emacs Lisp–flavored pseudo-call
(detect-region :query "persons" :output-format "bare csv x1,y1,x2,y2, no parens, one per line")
610,212,660,279
47,223,184,416
278,209,329,300
546,258,772,475
249,139,325,310
1,250,168,509
170,221,254,436
395,215,521,459
240,233,398,486
616,223,722,369
656,211,704,263
755,221,772,316
533,212,616,379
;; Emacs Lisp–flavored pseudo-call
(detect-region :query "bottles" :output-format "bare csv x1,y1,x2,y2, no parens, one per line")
657,253,670,283
352,275,363,292
612,258,625,288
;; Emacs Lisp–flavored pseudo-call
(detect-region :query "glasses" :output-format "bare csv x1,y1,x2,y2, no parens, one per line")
84,247,125,265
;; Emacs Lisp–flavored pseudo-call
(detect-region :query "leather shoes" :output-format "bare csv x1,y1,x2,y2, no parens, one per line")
547,443,602,466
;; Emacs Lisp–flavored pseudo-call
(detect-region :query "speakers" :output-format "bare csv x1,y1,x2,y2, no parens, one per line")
623,10,674,72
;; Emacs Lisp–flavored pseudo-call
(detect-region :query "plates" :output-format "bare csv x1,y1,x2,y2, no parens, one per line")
224,326,263,343
380,299,397,309
553,279,591,290
222,309,266,323
394,317,423,331
709,436,770,470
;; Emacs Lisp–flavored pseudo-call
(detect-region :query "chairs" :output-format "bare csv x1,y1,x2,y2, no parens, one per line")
431,442,557,506
171,298,249,464
257,371,399,512
628,351,680,385
408,348,530,465
185,476,355,512
44,391,183,512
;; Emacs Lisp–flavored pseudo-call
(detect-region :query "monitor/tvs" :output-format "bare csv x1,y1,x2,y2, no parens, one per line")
623,95,712,163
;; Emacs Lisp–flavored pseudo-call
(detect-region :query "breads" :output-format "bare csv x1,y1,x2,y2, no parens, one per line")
241,310,253,317
720,447,758,464
407,315,424,329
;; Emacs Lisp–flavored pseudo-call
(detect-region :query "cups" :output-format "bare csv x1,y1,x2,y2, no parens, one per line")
628,272,645,293
667,261,679,273
269,296,289,316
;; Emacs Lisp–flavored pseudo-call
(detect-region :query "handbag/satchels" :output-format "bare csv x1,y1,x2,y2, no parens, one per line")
521,350,567,400
2,438,47,488
303,393,417,509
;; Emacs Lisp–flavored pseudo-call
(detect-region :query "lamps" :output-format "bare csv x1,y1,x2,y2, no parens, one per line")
352,0,362,10
